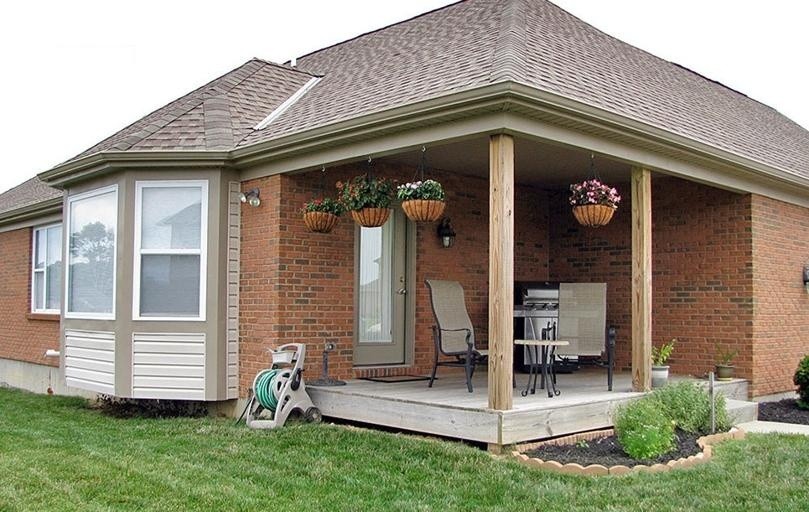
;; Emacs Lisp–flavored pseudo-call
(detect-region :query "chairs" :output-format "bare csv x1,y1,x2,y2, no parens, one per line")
424,279,515,392
539,281,618,390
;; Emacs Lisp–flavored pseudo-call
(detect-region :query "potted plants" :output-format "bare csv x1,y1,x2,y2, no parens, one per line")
651,338,677,386
713,340,740,381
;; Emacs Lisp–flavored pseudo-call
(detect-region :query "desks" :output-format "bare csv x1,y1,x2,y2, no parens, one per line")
512,337,571,398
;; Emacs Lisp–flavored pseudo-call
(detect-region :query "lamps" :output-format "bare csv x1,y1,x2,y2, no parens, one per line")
436,216,457,250
237,188,263,208
801,262,809,282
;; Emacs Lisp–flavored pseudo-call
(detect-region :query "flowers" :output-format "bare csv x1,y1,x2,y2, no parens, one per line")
334,174,398,210
299,197,346,217
396,178,447,201
568,179,622,210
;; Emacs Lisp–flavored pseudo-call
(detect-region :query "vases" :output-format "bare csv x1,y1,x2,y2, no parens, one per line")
401,199,446,224
349,207,394,227
570,203,616,229
302,211,341,233
